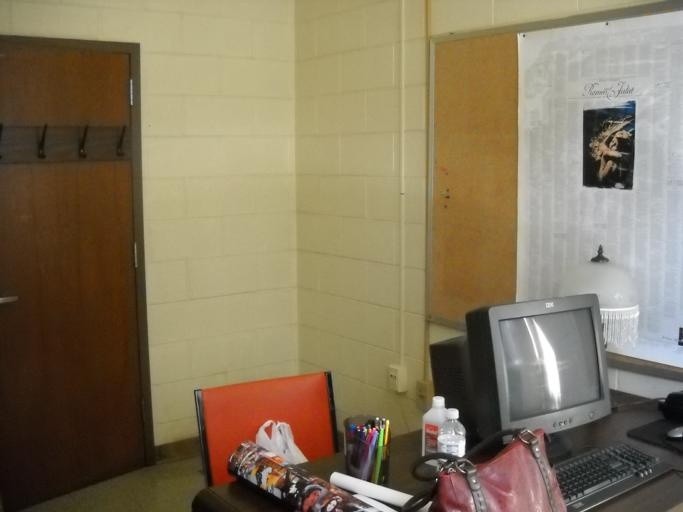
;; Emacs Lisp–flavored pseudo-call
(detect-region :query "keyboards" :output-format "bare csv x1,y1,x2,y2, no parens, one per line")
551,436,674,512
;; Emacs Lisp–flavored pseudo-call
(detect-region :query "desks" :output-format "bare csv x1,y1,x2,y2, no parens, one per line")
189,385,682,511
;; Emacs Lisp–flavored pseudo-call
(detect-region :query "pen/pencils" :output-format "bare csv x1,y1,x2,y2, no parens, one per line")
345,417,391,485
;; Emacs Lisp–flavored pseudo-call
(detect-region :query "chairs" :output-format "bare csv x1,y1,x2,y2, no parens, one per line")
190,369,339,485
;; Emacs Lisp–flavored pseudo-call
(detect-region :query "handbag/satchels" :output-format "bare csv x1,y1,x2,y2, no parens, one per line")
401,428,568,512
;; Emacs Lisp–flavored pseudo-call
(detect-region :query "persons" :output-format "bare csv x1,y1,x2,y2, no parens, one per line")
599,130,632,181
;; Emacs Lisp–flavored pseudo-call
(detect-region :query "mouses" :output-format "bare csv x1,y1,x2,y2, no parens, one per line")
667,425,683,439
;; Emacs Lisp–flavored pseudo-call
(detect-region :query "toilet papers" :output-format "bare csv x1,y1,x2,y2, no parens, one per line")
330,471,433,512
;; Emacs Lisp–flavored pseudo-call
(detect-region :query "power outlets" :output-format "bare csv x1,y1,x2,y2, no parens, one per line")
384,363,407,393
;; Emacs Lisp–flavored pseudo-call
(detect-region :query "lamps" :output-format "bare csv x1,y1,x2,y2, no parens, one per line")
572,243,644,352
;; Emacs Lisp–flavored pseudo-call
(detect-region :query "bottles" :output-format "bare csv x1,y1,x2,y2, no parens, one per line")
422,397,446,466
438,408,465,463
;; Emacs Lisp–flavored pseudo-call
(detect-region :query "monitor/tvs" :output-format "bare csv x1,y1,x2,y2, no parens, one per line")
427,292,614,447
